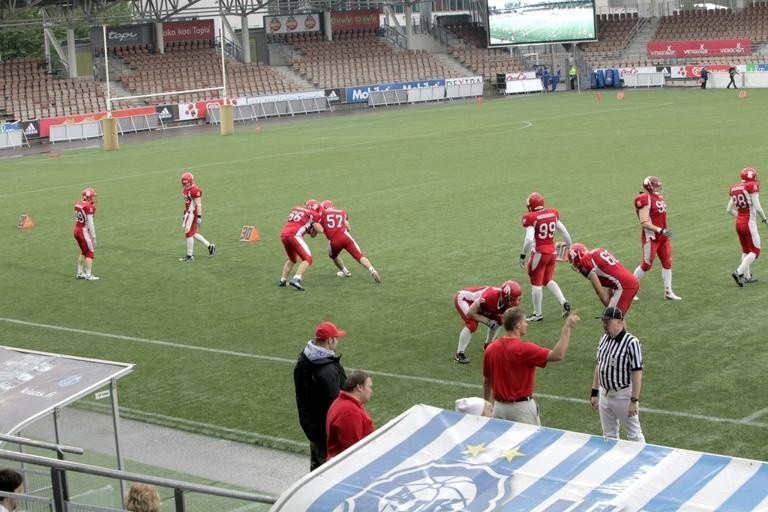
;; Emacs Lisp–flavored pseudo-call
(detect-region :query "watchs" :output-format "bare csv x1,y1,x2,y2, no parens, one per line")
631,397,639,402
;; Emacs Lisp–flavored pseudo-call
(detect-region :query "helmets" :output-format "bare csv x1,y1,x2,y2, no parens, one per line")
643,176,661,195
82,188,96,204
305,199,334,211
182,173,193,186
501,280,522,306
527,193,544,211
741,167,758,182
568,243,588,265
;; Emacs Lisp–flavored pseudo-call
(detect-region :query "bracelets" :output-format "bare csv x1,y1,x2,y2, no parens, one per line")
520,254,526,259
196,215,201,218
591,389,598,397
660,228,664,234
762,219,767,223
184,209,186,214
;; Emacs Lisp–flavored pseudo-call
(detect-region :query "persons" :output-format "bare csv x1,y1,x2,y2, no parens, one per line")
726,167,768,287
567,243,639,318
590,306,644,443
481,306,580,426
519,192,572,322
0,469,24,512
125,483,162,512
701,67,709,89
727,65,739,89
294,322,348,473
535,63,577,92
325,370,374,462
74,188,100,281
279,199,324,291
179,172,216,262
311,200,381,283
632,175,682,301
455,280,521,364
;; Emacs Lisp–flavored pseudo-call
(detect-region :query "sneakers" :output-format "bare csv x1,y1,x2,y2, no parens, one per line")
208,244,215,255
561,301,571,318
280,277,304,290
732,270,757,287
76,272,99,280
179,255,193,261
337,271,351,277
527,314,543,321
372,271,380,282
455,353,469,363
664,291,681,300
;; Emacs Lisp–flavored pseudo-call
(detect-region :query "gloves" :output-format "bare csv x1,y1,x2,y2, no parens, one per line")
657,228,672,238
197,215,201,224
520,254,525,268
486,319,499,329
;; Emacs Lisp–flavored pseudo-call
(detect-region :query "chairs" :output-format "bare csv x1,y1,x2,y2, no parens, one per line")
0,2,768,140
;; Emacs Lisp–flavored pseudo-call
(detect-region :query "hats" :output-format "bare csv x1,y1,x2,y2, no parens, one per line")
595,306,624,319
316,322,345,339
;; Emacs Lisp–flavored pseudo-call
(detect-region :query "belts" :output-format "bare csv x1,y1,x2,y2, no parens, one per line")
497,397,528,402
